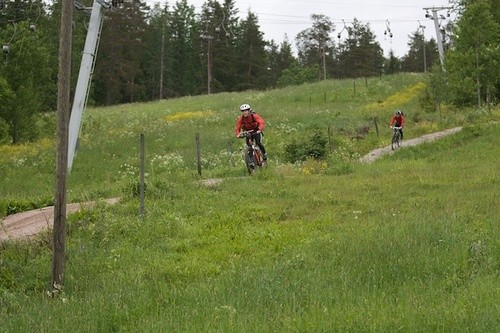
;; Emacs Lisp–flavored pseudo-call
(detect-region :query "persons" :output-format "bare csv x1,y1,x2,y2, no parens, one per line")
390,110,404,143
236,104,267,169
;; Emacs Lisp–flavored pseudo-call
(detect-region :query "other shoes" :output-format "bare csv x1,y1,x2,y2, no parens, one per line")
263,153,267,160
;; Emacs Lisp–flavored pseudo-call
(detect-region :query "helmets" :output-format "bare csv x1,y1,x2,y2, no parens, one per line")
240,104,250,111
397,111,401,115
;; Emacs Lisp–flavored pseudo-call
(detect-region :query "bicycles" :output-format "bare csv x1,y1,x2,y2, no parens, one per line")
236,129,268,174
389,126,401,150
